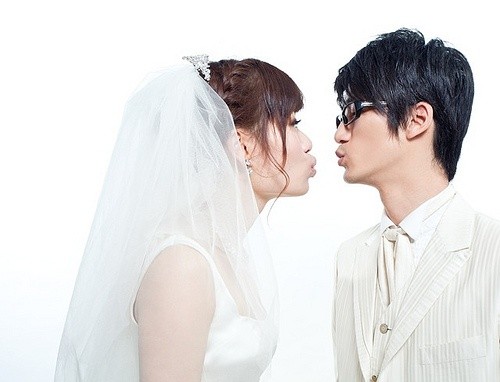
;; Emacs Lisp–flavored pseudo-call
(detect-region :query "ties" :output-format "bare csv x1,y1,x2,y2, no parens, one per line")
377,228,411,307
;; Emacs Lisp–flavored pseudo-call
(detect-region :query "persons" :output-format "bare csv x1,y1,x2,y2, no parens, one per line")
317,27,500,381
54,54,317,382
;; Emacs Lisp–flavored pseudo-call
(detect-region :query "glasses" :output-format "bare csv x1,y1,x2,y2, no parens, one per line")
335,99,422,127
